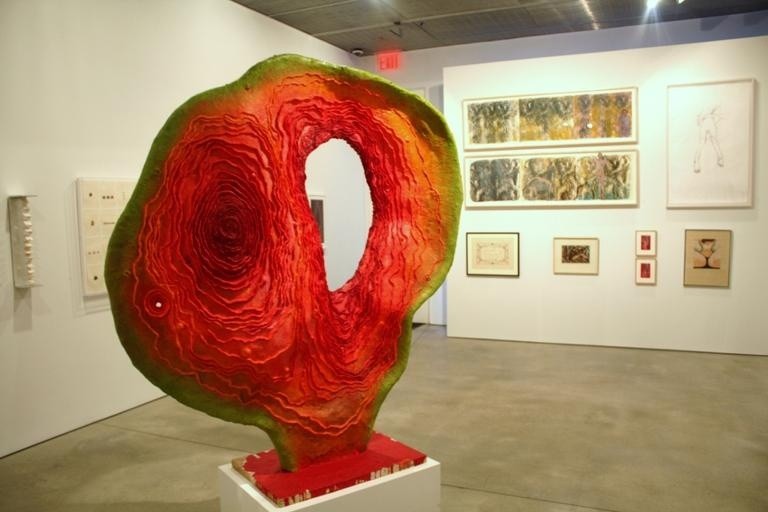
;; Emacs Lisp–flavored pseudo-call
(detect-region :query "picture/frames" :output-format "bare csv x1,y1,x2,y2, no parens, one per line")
309,195,327,247
636,231,656,255
463,86,639,150
76,177,138,297
465,149,640,210
553,238,600,276
684,228,732,289
635,258,657,286
466,232,520,276
666,81,754,210
